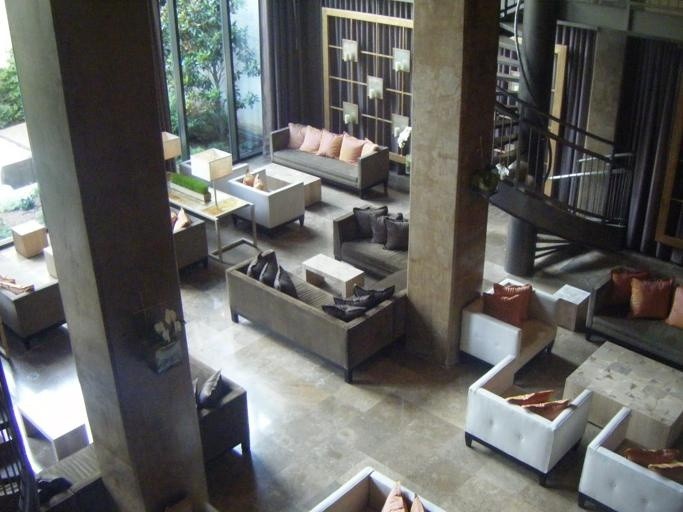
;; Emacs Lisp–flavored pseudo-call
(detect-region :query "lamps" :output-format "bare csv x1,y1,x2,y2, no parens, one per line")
190,147,231,207
160,131,180,161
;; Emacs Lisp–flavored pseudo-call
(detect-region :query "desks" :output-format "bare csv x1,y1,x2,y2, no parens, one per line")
164,177,259,265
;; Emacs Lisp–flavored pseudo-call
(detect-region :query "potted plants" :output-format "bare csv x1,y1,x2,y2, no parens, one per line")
169,173,211,203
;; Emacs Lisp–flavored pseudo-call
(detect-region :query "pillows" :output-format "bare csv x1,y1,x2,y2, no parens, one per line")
253,174,266,191
378,480,409,511
498,387,554,406
409,491,428,511
492,280,533,322
0,472,74,512
286,122,378,166
627,275,676,319
2,284,34,296
622,446,681,470
243,168,253,186
609,266,649,308
663,283,683,329
481,291,524,329
192,373,198,409
506,282,535,305
647,460,683,485
173,207,190,232
520,398,570,423
0,273,17,287
244,206,406,322
198,369,226,410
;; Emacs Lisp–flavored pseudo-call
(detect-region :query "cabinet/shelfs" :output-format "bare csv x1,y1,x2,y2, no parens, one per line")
551,283,592,332
9,219,49,263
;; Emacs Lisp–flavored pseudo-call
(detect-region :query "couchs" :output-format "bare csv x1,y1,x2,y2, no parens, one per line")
270,124,388,202
581,263,683,371
575,405,683,512
178,158,249,193
0,269,66,348
187,350,250,466
461,353,593,487
307,464,450,512
457,277,559,381
0,442,108,512
224,206,409,384
169,206,208,289
228,168,304,235
